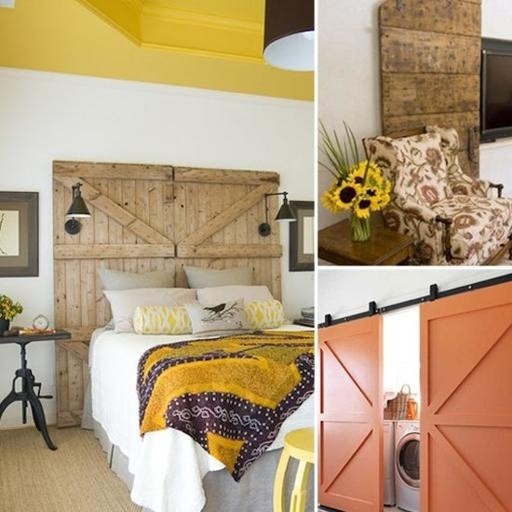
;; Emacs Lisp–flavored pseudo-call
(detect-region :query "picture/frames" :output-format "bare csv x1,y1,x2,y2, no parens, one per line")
0,191,39,278
289,201,316,271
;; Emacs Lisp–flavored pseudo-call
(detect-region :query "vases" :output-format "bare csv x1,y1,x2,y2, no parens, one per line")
0,317,10,337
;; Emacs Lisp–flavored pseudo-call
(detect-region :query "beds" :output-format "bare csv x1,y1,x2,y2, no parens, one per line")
81,265,313,512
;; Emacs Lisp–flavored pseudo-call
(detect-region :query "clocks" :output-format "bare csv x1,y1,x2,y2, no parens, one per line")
32,314,50,332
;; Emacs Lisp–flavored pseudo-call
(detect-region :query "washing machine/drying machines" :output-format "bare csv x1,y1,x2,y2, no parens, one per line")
394,420,421,512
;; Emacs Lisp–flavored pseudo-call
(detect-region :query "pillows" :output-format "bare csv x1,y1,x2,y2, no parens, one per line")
183,265,252,288
244,300,285,329
184,297,255,338
133,306,191,335
196,285,274,301
97,264,176,330
102,288,198,334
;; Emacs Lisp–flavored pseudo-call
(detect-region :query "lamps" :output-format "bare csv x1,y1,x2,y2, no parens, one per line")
258,192,297,238
263,1,316,72
63,182,92,234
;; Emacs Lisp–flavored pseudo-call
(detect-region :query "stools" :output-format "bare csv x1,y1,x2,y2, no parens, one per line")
273,427,315,511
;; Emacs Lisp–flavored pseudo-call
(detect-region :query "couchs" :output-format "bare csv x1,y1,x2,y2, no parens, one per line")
362,124,512,265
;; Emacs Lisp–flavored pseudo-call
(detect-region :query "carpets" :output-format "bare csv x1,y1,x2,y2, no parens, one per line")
0,423,143,512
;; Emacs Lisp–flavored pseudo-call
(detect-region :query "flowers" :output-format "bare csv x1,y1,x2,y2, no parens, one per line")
0,294,23,322
317,119,392,242
